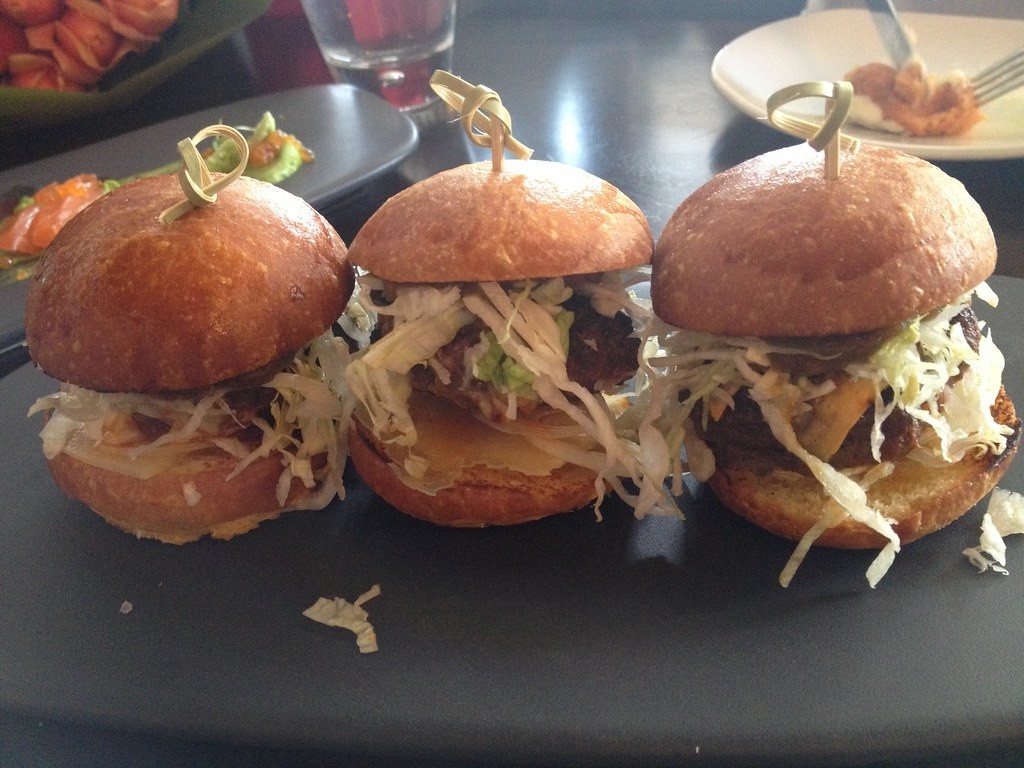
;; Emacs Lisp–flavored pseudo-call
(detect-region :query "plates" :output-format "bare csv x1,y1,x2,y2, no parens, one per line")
0,275,1023,767
0,1,271,128
710,5,1023,163
2,84,424,382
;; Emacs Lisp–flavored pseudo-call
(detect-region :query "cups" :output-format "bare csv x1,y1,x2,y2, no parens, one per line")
299,0,454,112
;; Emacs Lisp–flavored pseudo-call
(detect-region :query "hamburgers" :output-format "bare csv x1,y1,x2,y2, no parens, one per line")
28,80,1022,595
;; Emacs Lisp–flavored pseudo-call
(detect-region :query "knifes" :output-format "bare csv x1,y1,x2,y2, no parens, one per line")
866,1,915,73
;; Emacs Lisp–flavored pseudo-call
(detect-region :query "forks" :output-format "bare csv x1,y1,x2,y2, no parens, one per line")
969,48,1024,108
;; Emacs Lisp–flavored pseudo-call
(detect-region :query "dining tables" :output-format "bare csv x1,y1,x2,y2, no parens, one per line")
2,20,1024,768
